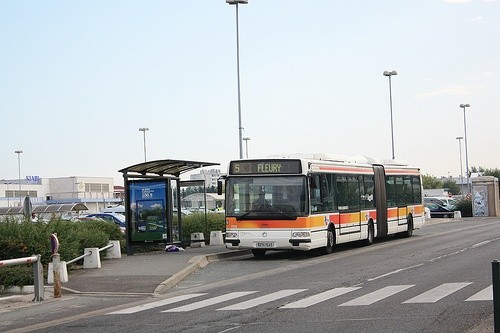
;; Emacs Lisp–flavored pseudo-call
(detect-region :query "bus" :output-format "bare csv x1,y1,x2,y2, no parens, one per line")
217,157,424,258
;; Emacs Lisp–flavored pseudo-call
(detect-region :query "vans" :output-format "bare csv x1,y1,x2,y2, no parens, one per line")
103,205,143,219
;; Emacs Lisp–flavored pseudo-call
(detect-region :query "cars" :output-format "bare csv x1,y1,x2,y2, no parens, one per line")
83,212,147,234
422,197,463,218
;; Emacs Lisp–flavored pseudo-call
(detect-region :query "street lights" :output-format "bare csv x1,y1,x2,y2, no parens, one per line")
139,127,149,163
226,0,252,159
459,104,470,192
383,70,398,160
456,137,465,195
14,150,23,207
